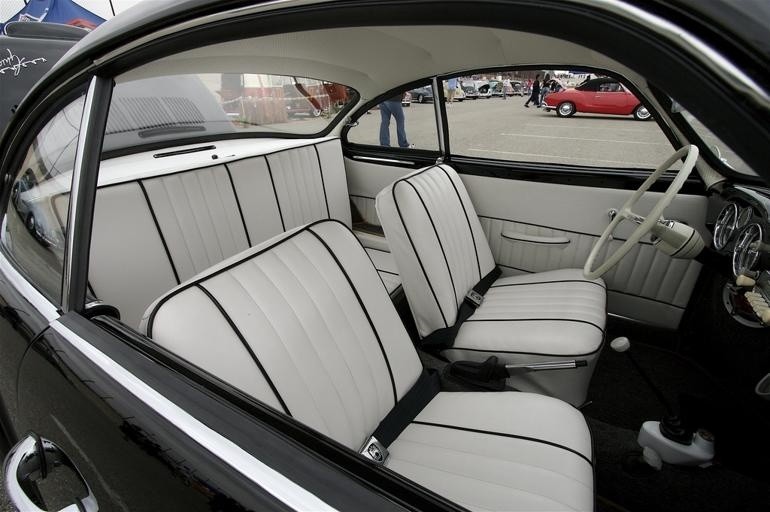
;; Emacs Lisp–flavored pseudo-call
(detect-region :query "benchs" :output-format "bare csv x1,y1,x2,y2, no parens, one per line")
44,134,402,332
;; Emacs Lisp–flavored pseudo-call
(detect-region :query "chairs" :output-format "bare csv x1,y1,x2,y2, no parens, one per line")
375,165,607,408
140,218,598,512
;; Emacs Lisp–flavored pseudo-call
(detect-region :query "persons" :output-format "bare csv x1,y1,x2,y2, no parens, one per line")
554,85,562,92
580,74,591,85
520,80,527,98
445,78,459,105
551,81,558,92
524,73,541,109
378,92,417,150
502,76,510,100
528,79,532,95
539,74,551,105
